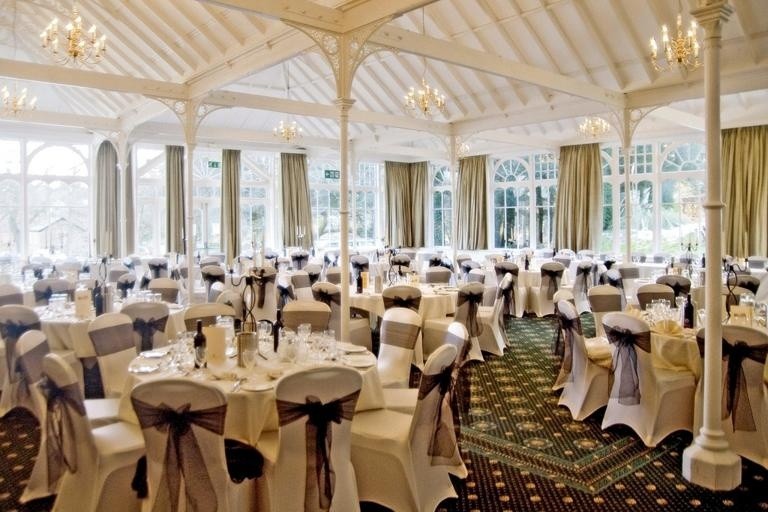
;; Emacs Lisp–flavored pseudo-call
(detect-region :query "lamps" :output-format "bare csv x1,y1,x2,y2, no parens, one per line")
579,116,611,140
41,0,107,71
273,86,303,142
404,5,448,120
453,141,470,159
649,0,705,79
0,0,36,119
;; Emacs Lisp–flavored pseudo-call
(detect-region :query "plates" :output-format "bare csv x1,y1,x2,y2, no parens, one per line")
127,344,377,393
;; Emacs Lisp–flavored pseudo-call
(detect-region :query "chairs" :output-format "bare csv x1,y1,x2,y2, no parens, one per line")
43,353,147,512
14,330,122,503
347,343,459,512
258,368,364,512
130,377,256,512
380,321,472,483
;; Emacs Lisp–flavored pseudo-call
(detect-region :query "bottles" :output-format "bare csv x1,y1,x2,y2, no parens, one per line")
357,270,363,294
273,309,284,352
525,255,529,270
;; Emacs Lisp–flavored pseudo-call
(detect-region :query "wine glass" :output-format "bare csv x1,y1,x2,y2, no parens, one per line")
166,316,338,380
633,254,641,263
646,267,768,332
48,288,162,314
